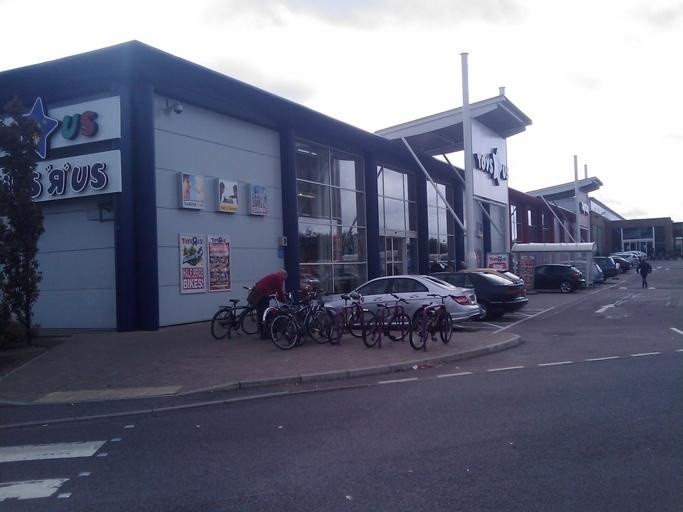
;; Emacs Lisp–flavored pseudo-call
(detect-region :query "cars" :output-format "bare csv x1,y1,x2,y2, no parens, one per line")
318,272,481,333
296,257,462,299
429,247,648,325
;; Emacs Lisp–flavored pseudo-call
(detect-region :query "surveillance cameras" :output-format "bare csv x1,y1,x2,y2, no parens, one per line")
173,102,184,114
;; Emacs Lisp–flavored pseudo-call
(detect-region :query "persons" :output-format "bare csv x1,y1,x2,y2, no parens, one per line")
255,269,288,339
637,260,648,288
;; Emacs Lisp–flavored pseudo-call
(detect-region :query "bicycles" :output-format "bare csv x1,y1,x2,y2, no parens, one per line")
210,279,457,355
